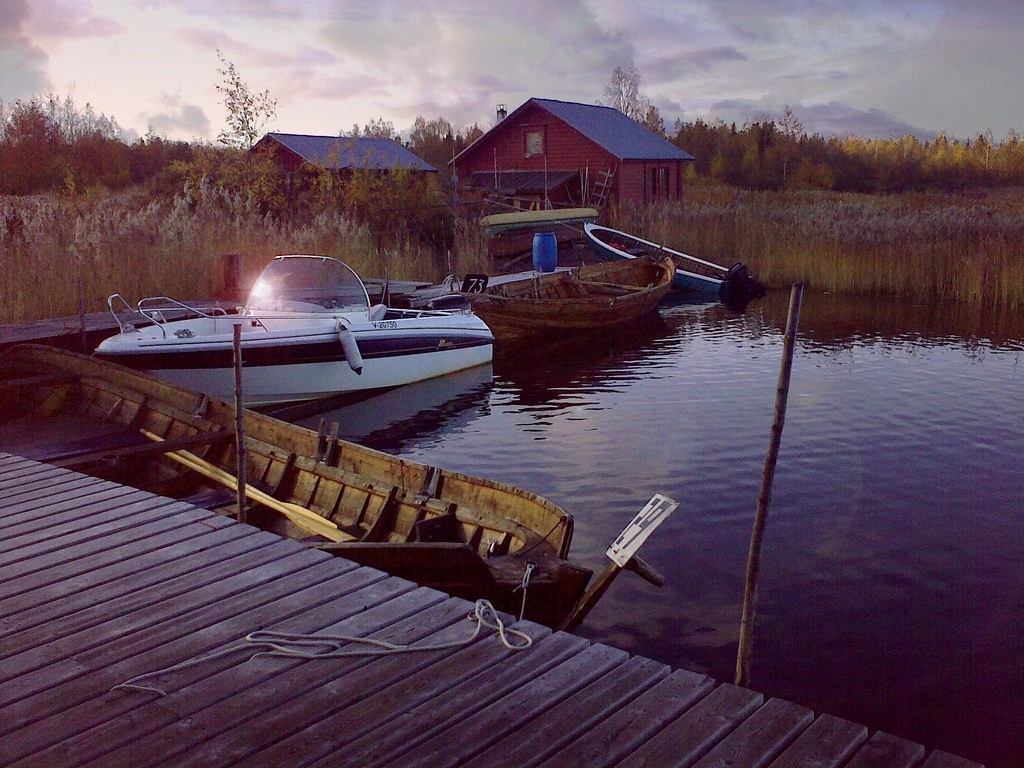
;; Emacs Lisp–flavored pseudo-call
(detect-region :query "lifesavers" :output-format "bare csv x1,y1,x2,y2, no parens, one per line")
428,294,467,311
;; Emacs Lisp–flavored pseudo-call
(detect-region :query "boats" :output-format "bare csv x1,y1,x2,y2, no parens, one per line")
91,253,496,412
0,340,597,611
583,221,766,302
426,250,676,348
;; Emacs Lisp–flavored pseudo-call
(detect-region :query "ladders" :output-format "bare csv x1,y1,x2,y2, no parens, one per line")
587,159,618,214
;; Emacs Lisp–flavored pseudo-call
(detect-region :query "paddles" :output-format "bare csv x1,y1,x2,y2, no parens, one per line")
163,451,357,543
139,427,337,536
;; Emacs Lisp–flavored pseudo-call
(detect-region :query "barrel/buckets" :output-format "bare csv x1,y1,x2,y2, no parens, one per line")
533,231,557,273
